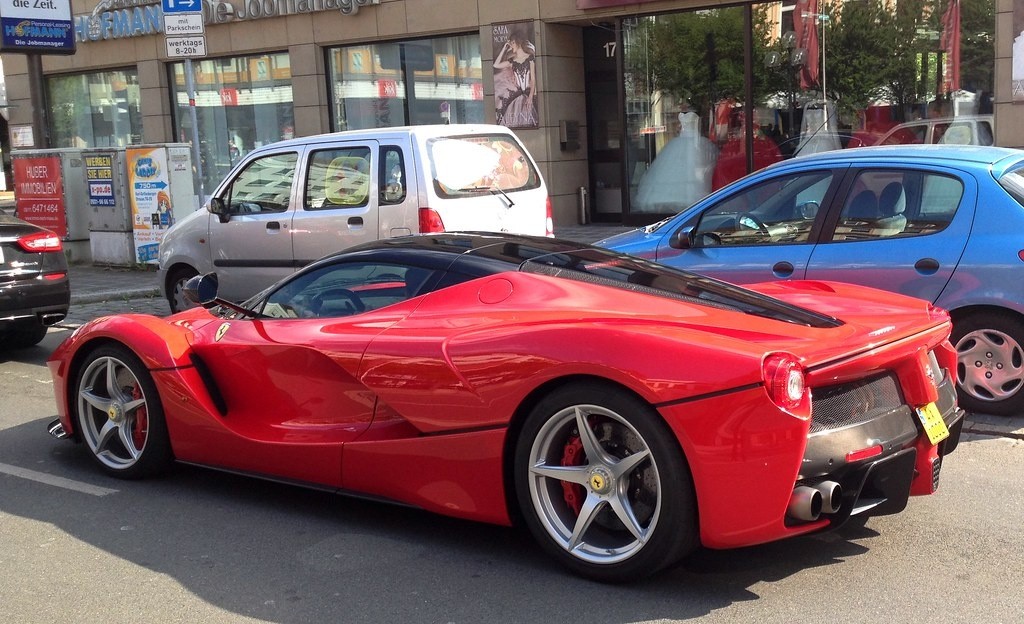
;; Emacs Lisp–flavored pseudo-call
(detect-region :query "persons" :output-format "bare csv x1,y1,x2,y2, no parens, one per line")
494,31,535,122
653,91,991,209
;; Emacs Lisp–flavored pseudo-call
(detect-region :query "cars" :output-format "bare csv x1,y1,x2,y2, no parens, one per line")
587,145,1023,418
0,205,72,350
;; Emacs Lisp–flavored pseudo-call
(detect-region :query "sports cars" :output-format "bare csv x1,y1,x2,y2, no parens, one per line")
46,231,967,590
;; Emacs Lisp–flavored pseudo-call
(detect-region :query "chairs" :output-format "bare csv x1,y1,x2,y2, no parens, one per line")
842,181,906,238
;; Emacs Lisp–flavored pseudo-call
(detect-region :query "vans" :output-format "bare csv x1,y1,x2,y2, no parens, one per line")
782,114,998,223
157,125,553,315
